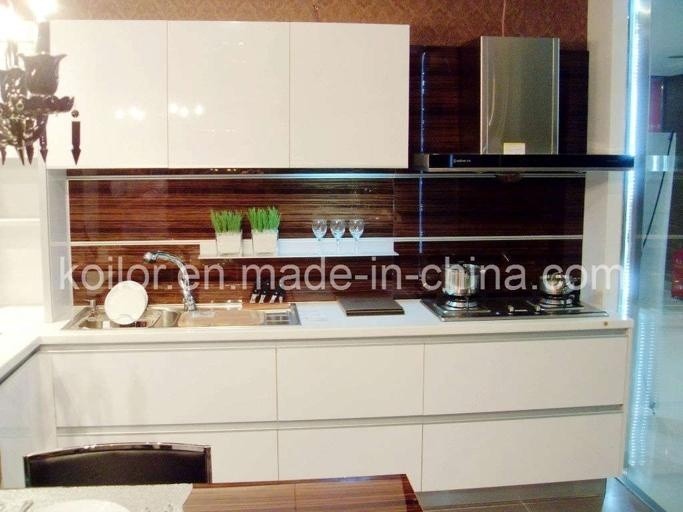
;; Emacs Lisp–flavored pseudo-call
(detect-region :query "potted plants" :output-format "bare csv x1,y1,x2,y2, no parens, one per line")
207,202,246,257
247,201,280,253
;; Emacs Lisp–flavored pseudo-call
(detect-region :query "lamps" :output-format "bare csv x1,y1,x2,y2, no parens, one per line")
1,0,85,166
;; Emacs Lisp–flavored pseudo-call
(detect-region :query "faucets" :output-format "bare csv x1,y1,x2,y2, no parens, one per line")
142,251,197,312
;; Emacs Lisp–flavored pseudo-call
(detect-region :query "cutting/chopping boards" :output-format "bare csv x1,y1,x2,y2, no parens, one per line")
241,293,292,310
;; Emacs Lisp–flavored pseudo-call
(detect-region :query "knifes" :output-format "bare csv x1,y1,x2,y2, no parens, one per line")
250,281,289,304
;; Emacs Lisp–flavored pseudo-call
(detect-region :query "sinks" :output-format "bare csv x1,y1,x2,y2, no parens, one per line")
61,305,179,328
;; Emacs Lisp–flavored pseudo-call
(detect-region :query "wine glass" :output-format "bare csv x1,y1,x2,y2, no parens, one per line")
346,216,365,255
328,217,349,257
309,217,327,256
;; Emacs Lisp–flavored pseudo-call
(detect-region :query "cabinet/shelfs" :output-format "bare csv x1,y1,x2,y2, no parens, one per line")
49,16,168,171
167,19,411,176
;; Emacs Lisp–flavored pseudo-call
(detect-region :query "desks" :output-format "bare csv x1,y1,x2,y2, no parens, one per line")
0,473,424,512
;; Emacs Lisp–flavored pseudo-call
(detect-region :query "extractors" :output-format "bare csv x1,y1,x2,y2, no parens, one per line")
415,34,636,176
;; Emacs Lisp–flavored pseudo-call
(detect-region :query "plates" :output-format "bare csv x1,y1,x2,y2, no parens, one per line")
104,279,150,325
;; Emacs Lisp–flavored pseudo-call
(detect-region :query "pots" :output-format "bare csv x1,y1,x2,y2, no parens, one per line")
538,270,577,296
438,259,478,298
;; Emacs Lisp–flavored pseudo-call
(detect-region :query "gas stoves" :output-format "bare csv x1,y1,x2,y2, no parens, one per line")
417,294,611,324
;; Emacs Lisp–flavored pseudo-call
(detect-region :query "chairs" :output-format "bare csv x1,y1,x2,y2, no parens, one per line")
19,441,213,489
